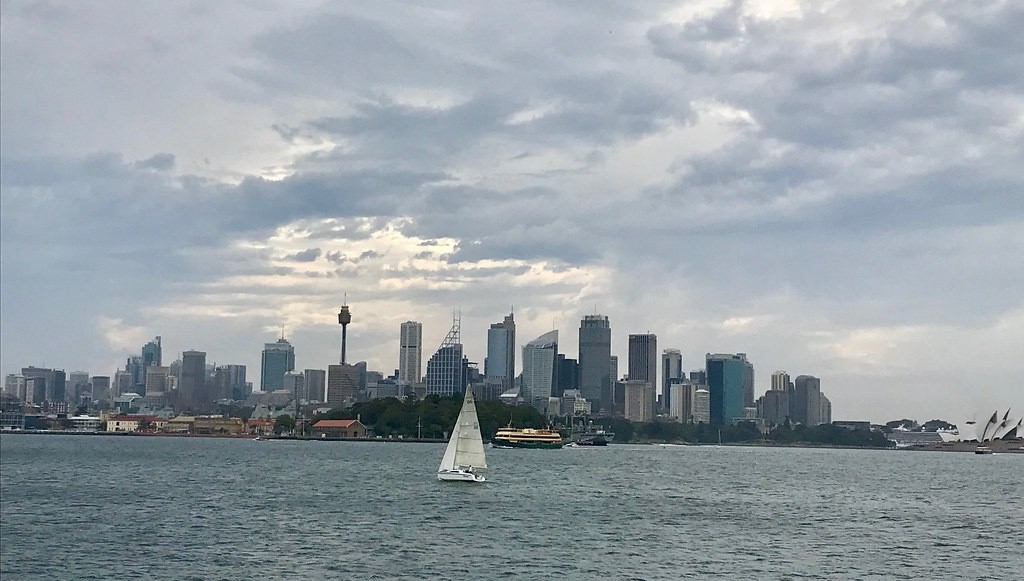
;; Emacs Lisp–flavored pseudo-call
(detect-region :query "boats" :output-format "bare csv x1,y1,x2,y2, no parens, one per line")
490,427,562,449
574,420,616,446
975,446,992,454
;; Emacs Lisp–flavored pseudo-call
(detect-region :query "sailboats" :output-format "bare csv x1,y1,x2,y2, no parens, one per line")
437,382,488,483
